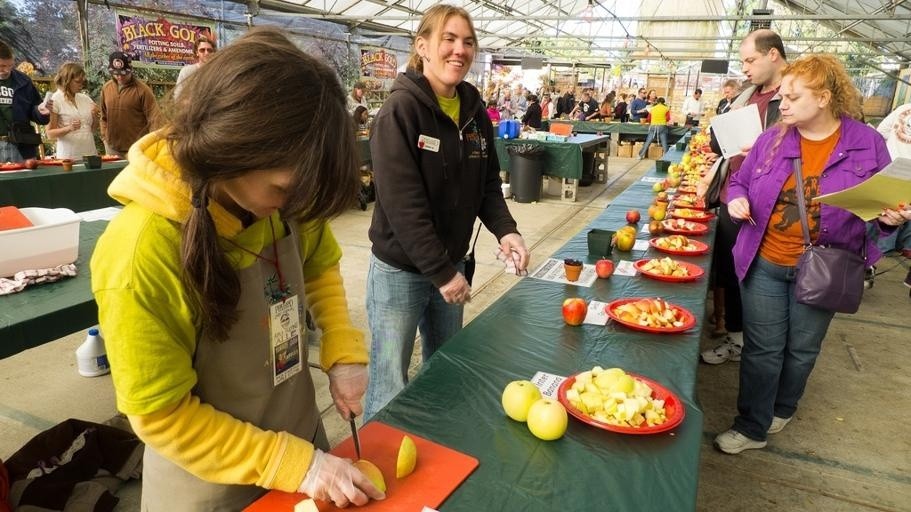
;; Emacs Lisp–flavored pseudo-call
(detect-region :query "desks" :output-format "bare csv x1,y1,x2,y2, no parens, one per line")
0,219,110,360
571,120,719,186
357,132,610,189
359,186,719,512
0,160,130,213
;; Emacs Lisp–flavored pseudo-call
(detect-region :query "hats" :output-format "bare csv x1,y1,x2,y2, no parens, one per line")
108,51,133,76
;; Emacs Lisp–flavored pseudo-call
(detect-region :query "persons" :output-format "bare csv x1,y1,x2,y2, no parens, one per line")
363,3,530,427
346,82,368,117
700,29,791,367
39,63,101,161
99,51,163,156
683,89,705,126
89,26,385,512
173,37,217,104
715,80,740,114
714,53,911,455
875,102,911,163
0,41,54,163
350,106,371,136
486,84,670,160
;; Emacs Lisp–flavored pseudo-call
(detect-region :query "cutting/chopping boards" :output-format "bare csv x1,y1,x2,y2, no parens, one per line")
243,418,482,512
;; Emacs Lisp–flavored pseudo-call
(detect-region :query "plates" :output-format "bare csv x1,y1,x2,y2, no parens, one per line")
661,219,710,234
633,258,706,281
103,155,123,162
41,157,74,165
605,296,698,334
559,369,687,434
669,137,719,221
1,161,23,172
648,236,711,257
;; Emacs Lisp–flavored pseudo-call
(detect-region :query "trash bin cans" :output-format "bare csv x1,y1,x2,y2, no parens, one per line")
505,143,546,203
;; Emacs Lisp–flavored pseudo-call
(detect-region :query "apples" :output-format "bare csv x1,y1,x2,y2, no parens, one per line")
502,379,542,421
596,256,614,279
527,400,568,441
25,160,37,170
614,182,668,251
563,298,586,326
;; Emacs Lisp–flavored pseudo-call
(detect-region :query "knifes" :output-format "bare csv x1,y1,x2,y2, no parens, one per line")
346,407,366,463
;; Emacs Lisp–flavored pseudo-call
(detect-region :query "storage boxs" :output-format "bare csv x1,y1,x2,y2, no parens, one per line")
0,206,83,279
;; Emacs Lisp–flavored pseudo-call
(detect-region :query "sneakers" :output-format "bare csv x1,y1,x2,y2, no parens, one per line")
699,338,745,366
714,428,768,455
765,415,792,434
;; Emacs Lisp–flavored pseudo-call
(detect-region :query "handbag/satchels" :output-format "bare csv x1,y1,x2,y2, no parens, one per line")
794,247,867,314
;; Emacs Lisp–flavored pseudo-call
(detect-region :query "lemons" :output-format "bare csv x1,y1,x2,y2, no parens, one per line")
396,435,417,478
351,459,386,493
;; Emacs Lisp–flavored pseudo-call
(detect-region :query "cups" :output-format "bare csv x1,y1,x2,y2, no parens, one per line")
564,261,583,282
574,132,578,137
674,122,678,127
62,158,72,171
691,129,698,136
640,118,647,124
605,117,611,122
657,160,668,172
587,228,618,256
597,130,603,136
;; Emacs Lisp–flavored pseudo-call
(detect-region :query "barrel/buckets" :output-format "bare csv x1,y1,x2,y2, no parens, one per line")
501,183,512,199
498,119,520,141
